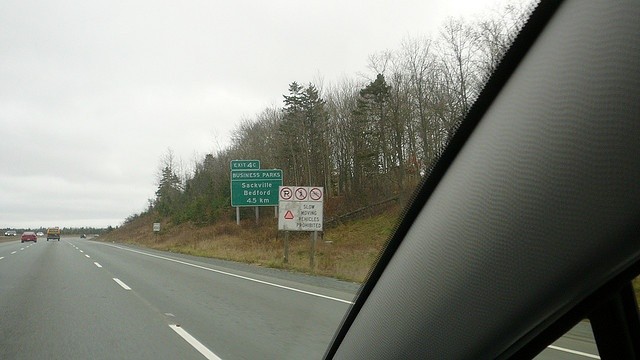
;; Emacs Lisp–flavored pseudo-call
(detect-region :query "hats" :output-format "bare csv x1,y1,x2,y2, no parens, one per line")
21,232,37,243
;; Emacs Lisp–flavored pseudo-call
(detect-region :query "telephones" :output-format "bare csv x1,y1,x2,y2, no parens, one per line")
230,160,283,207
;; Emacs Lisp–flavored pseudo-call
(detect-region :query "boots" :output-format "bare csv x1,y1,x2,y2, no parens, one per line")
47,228,60,241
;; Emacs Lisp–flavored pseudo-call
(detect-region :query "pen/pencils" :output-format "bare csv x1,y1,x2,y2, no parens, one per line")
5,231,17,236
